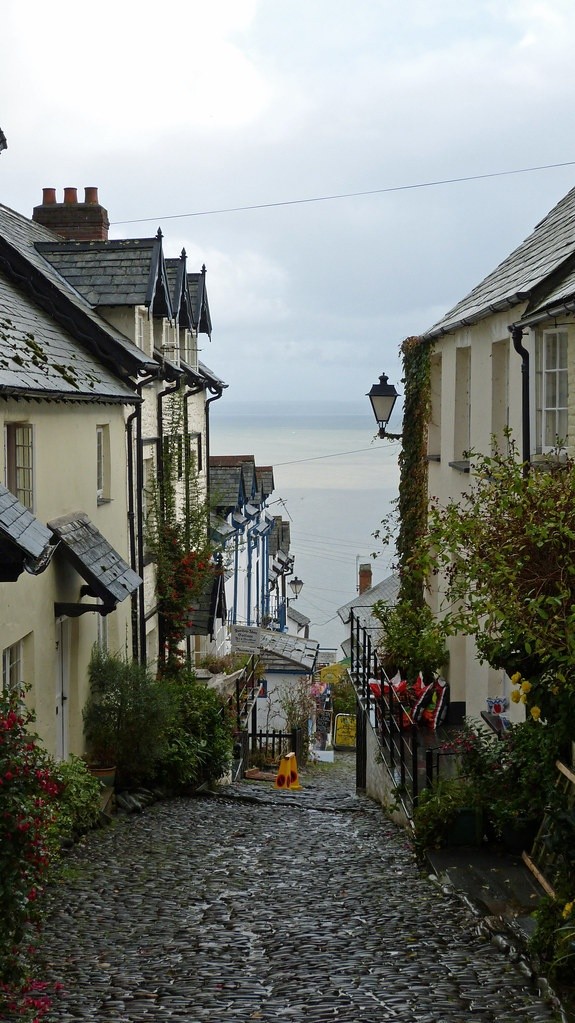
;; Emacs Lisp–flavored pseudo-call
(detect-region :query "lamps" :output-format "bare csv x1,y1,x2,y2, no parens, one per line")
286,576,305,608
365,372,406,440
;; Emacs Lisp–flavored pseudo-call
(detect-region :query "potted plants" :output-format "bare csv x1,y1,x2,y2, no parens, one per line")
73,684,118,784
372,596,450,688
414,713,565,857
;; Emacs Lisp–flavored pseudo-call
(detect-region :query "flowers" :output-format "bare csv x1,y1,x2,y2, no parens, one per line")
430,720,515,770
158,520,209,649
